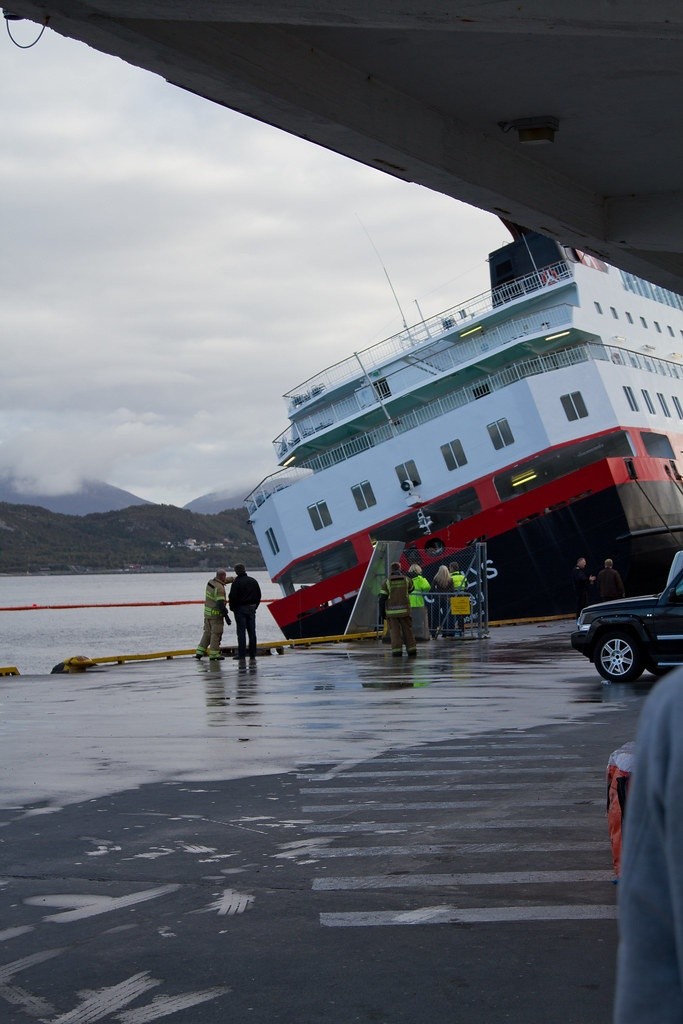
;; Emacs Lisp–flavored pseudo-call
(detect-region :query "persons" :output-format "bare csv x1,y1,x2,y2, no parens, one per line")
228,564,262,659
428,565,455,639
376,561,418,658
443,561,469,637
597,558,625,603
608,664,682,1022
403,564,434,642
194,569,237,662
571,556,596,617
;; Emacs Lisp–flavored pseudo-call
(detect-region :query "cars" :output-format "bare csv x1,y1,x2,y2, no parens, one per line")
570,564,683,681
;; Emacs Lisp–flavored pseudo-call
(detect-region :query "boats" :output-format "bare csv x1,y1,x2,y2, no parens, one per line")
242,209,683,643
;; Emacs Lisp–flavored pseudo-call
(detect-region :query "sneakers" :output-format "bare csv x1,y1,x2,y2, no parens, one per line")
209,656,225,660
194,654,204,660
232,655,245,660
250,656,256,659
